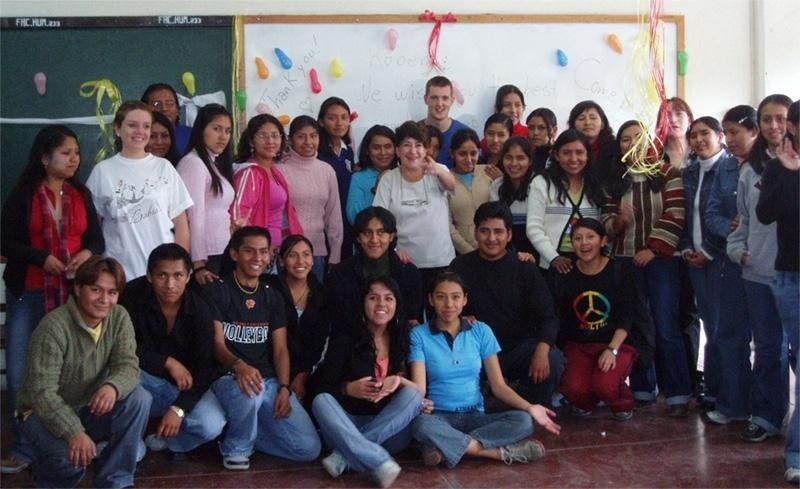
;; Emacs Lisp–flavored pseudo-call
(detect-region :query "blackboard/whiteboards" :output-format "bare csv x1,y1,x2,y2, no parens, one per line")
234,15,684,162
1,16,233,204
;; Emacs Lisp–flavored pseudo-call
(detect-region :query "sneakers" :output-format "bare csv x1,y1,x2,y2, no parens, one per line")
222,453,251,471
0,454,31,475
665,403,692,419
704,408,754,427
499,435,547,466
98,434,168,462
738,422,778,444
418,444,446,468
368,455,403,489
608,409,634,422
321,449,349,480
483,377,593,420
782,466,800,486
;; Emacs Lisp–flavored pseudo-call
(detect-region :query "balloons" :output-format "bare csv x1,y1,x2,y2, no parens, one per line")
309,68,322,93
678,52,688,77
255,57,269,79
557,50,567,67
33,71,47,96
387,29,398,50
607,34,623,52
182,71,196,96
274,47,292,69
329,56,342,78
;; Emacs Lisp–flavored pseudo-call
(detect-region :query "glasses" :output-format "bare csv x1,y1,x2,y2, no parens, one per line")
147,98,177,110
398,164,431,208
253,130,282,142
